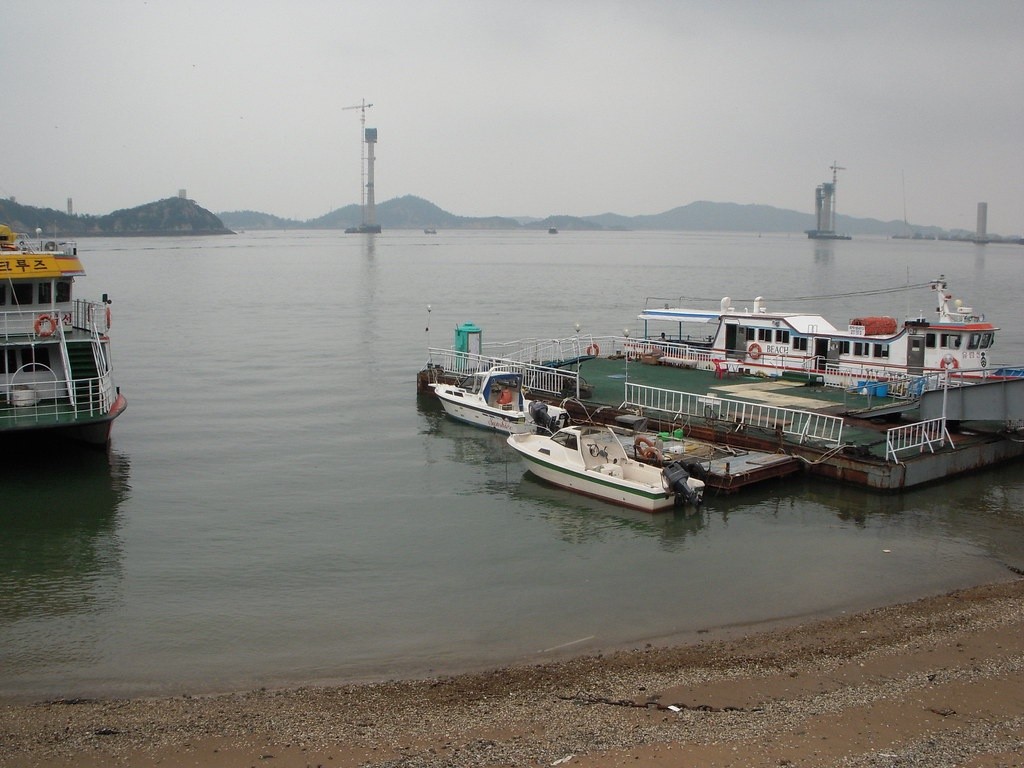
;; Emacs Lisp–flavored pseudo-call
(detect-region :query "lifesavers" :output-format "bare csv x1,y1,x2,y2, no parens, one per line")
748,343,761,359
587,343,599,357
940,358,958,374
35,314,56,337
107,307,111,328
635,437,653,457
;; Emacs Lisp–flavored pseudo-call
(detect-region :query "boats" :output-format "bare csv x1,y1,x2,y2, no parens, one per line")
620,267,1024,396
427,365,570,439
0,217,131,445
506,419,706,514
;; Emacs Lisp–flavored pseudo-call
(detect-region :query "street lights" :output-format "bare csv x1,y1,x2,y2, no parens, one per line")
623,328,631,383
425,305,434,364
574,322,582,372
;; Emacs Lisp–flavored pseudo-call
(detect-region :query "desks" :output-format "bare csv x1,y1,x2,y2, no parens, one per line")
718,362,745,379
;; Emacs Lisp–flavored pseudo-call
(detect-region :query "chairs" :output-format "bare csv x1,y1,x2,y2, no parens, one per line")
712,359,729,380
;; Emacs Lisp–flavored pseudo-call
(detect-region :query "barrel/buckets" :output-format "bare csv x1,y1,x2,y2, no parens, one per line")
857,380,888,398
674,428,683,438
658,432,670,441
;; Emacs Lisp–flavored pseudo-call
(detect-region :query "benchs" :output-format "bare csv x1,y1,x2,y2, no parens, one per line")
726,411,794,436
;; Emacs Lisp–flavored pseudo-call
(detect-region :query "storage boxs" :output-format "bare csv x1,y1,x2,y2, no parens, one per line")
644,355,657,365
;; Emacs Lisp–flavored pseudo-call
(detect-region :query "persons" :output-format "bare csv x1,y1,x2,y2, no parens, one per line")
656,332,667,341
497,387,512,404
